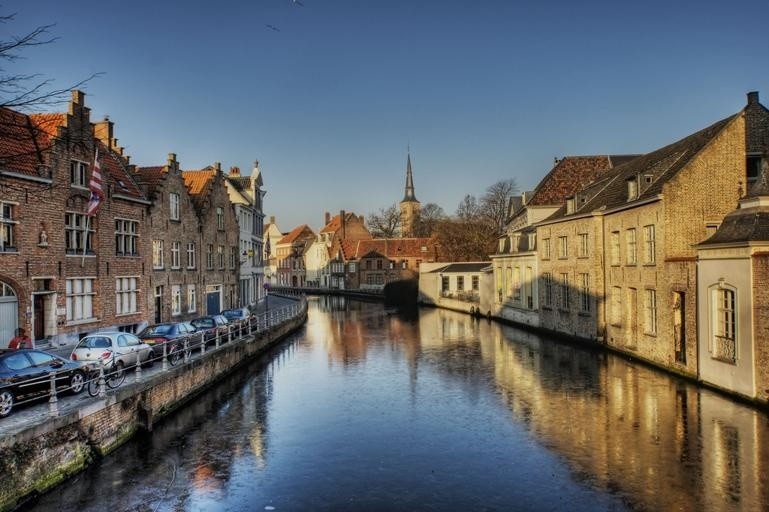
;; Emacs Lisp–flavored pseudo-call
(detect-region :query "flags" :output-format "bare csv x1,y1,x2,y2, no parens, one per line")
85,150,105,217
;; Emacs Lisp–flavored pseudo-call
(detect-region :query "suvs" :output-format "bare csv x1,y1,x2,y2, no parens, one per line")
191,315,236,345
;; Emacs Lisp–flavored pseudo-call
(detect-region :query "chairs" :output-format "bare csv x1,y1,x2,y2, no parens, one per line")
95,339,107,348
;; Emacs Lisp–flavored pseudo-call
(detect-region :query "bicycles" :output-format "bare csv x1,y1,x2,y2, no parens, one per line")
168,334,192,366
88,348,127,397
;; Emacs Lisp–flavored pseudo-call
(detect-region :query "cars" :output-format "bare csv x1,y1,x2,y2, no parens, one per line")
0,347,88,417
221,308,258,333
71,332,156,378
136,322,209,360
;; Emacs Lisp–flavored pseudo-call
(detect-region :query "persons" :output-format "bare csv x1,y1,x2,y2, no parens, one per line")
8,328,34,350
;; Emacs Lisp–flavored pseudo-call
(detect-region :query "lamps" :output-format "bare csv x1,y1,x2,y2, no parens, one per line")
238,250,248,266
